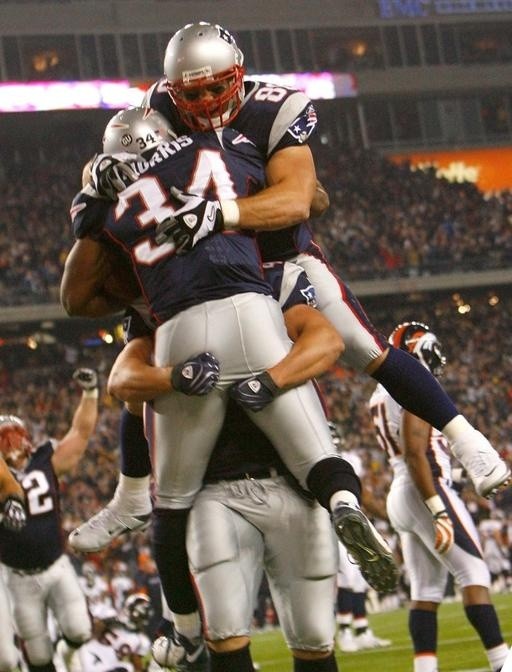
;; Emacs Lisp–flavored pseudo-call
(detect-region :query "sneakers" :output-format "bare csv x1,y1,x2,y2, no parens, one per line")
151,628,210,672
331,506,400,596
356,629,393,650
449,430,512,500
68,489,153,556
335,628,358,652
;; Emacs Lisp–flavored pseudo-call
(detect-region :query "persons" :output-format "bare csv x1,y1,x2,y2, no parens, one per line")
74,517,164,670
60,107,401,671
62,392,120,531
338,452,390,651
367,323,512,671
478,504,512,594
0,366,100,670
2,372,80,443
108,256,346,668
69,20,512,552
1,172,63,308
310,139,511,281
314,362,384,450
430,297,511,463
0,457,27,669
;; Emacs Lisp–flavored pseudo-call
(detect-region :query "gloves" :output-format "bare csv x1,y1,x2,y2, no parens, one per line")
171,352,220,397
424,495,454,556
3,500,27,533
227,371,282,414
72,367,99,399
154,186,240,260
89,153,141,203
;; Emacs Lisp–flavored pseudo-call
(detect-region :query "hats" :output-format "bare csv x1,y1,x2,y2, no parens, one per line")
82,563,95,576
112,562,128,571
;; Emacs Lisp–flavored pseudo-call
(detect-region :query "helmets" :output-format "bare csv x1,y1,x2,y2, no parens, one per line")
163,22,244,133
124,593,154,630
102,107,178,161
387,321,446,378
0,415,33,469
